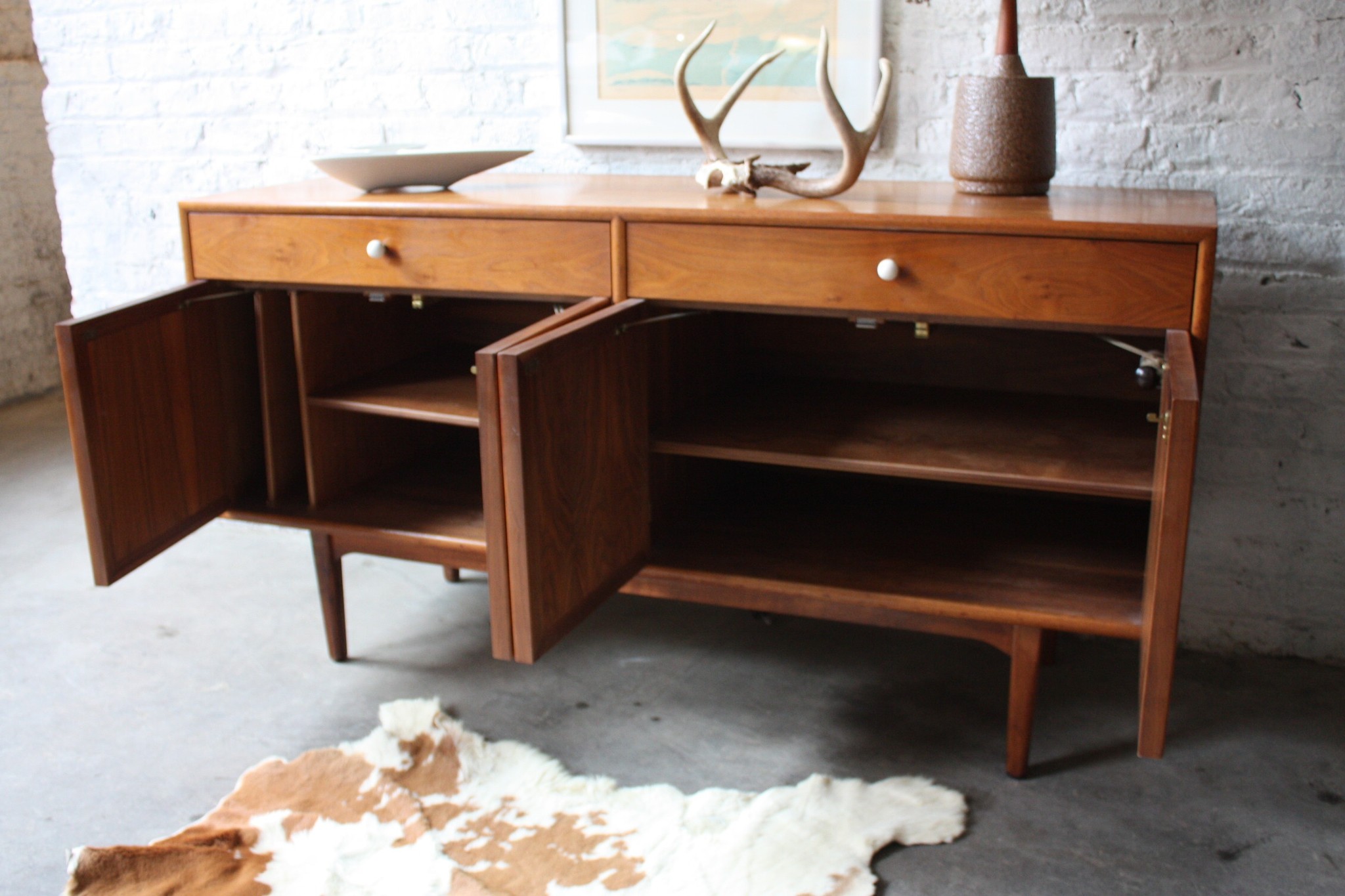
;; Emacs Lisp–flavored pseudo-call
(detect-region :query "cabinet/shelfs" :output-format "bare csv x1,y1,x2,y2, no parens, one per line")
54,173,1219,777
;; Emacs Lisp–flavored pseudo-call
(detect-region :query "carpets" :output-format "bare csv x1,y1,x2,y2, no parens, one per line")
65,697,964,896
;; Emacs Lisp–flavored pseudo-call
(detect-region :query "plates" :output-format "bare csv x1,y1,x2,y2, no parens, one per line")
312,148,533,193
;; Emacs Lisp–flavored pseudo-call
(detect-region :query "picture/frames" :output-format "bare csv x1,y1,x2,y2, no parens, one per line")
558,0,883,152
